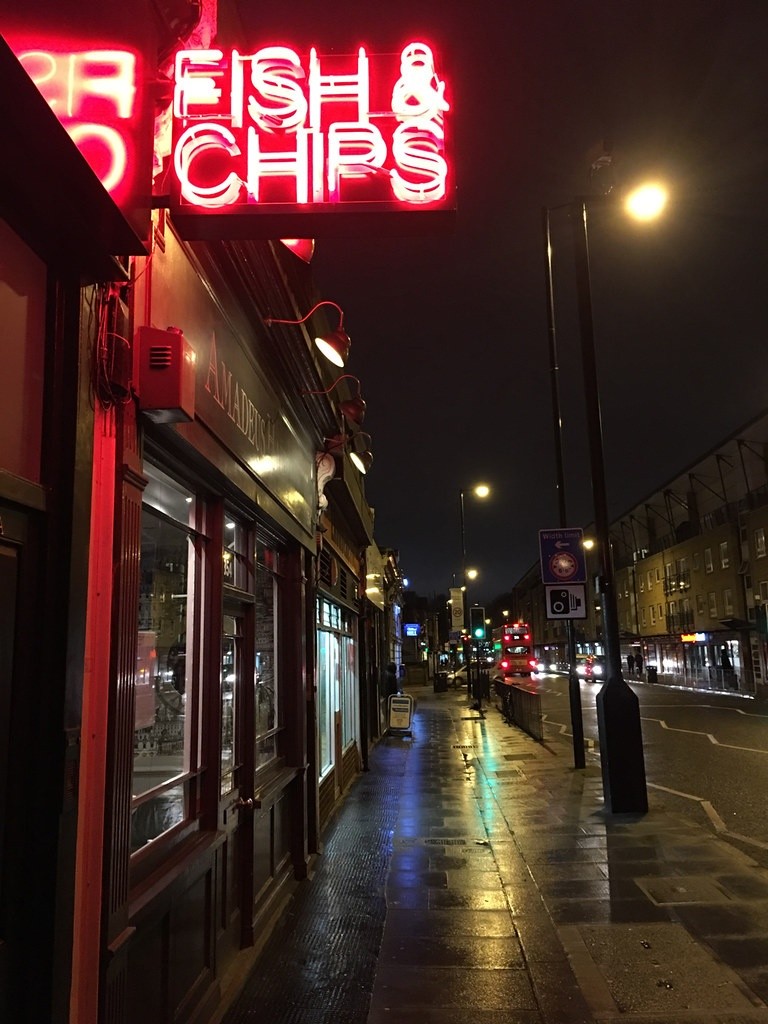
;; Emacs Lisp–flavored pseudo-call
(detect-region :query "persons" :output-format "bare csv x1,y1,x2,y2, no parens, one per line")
381,662,397,726
626,652,643,676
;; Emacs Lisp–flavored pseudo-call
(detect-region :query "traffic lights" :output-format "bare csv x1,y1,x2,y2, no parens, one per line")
469,607,485,639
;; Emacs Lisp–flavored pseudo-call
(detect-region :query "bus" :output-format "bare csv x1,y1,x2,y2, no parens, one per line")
492,623,533,678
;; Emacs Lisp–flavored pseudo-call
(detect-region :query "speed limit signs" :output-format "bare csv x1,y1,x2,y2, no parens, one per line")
453,607,462,617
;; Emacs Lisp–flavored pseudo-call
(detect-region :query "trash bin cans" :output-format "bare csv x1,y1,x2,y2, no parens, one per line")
433,672,448,693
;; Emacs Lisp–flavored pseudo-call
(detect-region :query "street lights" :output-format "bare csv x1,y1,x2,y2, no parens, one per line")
459,485,489,700
446,599,453,642
453,569,479,588
578,182,672,818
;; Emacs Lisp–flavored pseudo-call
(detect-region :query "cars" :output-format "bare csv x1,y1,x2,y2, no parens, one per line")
574,661,606,681
536,659,558,673
447,660,505,687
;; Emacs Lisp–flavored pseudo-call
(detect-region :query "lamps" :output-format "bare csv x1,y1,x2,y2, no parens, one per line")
264,302,352,368
322,431,376,475
300,375,367,426
279,238,315,264
